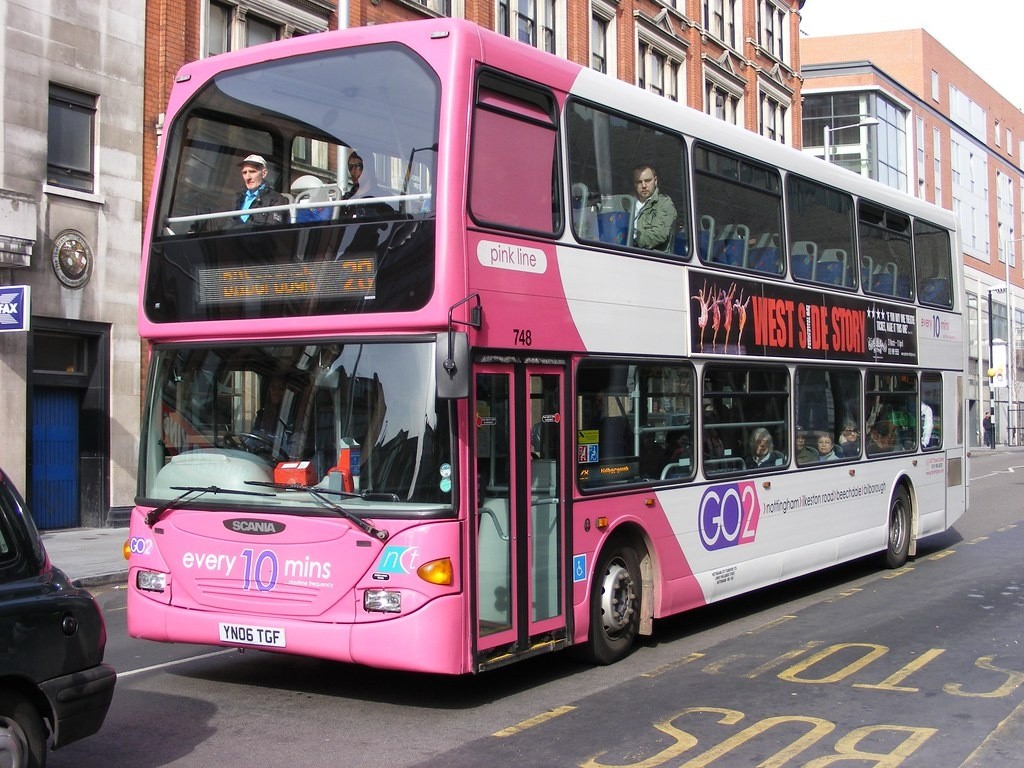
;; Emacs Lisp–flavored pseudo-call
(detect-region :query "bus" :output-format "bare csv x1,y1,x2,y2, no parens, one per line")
121,16,976,675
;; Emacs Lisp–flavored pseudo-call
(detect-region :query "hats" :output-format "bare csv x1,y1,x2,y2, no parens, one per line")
237,155,267,169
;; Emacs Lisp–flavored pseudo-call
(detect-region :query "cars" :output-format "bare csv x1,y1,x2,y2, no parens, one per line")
0,465,120,768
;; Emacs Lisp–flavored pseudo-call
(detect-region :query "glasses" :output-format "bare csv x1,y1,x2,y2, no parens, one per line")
347,163,365,171
268,383,285,390
844,428,858,435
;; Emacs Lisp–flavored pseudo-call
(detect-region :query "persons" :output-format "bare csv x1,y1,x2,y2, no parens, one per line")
240,374,315,455
742,427,779,468
165,155,286,236
815,434,838,461
899,389,933,448
531,421,542,452
642,430,728,473
984,412,992,446
361,379,439,503
796,425,818,463
342,149,399,214
629,166,677,251
839,420,859,457
867,417,896,450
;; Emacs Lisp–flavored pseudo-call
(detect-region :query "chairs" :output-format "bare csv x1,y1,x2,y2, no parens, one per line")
713,224,750,267
816,249,848,286
834,445,845,458
791,241,818,280
921,277,948,305
775,450,785,463
570,182,589,239
748,232,780,274
588,194,636,247
806,446,820,461
674,214,716,262
847,255,874,291
872,261,898,296
897,271,910,298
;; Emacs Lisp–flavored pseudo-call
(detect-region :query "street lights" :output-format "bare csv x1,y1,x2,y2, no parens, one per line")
988,369,996,449
